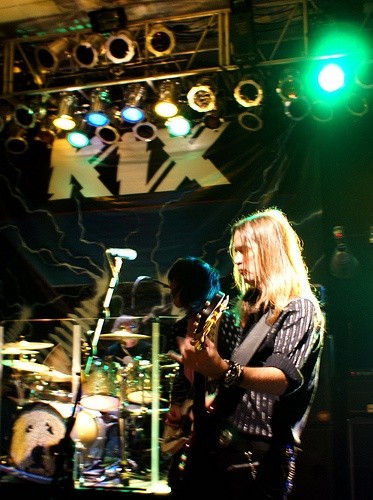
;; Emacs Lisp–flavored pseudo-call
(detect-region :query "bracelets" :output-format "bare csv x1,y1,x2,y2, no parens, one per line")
218,359,245,387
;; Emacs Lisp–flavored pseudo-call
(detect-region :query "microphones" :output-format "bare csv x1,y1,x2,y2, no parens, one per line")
106,247,138,260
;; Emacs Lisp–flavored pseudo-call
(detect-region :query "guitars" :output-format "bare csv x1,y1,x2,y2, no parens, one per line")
168,290,233,500
160,387,219,458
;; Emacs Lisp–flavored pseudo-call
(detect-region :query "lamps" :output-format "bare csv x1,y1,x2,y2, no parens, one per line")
0,6,373,156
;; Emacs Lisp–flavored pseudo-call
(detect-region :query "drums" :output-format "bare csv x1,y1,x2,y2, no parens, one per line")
40,390,72,403
9,400,106,479
124,360,161,404
80,358,123,414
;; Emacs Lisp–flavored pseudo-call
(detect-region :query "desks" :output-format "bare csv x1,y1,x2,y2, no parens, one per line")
301,421,336,500
345,415,373,499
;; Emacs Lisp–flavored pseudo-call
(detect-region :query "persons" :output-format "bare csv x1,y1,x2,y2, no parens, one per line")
110,209,326,500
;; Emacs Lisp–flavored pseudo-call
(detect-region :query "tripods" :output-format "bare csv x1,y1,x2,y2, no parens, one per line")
100,379,149,481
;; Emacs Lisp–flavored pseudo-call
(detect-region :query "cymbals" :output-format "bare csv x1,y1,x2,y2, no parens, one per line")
0,349,39,356
0,359,50,372
26,370,72,382
99,329,151,341
0,340,54,349
